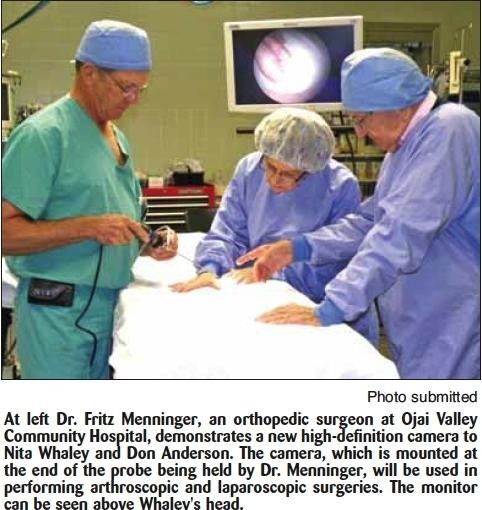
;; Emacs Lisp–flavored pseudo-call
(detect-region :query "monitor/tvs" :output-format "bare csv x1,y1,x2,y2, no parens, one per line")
2,80,12,138
223,15,364,113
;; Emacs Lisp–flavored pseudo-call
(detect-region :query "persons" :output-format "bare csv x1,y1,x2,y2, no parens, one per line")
1,18,481,380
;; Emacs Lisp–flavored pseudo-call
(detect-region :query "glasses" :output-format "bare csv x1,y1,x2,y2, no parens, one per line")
258,154,307,183
102,73,149,102
350,111,373,131
